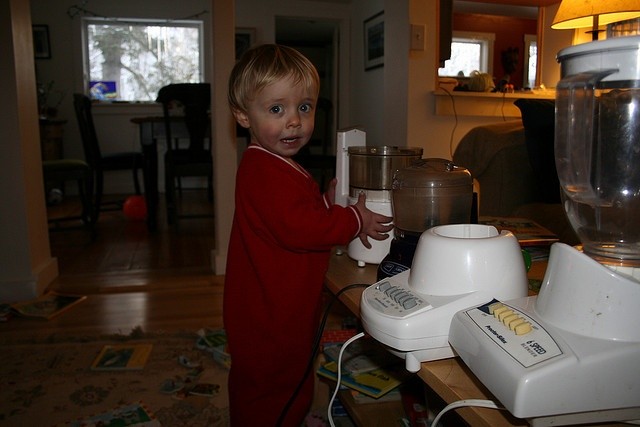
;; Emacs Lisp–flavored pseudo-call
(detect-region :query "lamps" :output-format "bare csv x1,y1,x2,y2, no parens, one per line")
552,1,640,41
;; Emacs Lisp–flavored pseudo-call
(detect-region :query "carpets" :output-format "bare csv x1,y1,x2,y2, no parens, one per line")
0,325,359,427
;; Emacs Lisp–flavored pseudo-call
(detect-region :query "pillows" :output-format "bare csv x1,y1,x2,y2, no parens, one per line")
514,96,569,205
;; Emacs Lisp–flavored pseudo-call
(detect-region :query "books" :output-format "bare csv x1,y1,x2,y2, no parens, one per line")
10,290,88,320
479,215,559,262
90,343,154,370
74,398,161,426
316,339,407,398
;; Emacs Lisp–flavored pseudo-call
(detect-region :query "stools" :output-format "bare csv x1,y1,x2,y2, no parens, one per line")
42,160,95,231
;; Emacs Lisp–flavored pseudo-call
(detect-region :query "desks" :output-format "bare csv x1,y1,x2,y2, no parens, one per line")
323,215,639,426
130,115,210,232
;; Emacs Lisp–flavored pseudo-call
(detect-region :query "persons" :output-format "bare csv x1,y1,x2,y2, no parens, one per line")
222,44,394,426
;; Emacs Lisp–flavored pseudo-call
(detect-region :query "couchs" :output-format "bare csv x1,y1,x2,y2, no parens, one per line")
451,119,576,232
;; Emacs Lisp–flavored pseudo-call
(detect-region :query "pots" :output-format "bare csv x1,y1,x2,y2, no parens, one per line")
347,143,425,268
376,157,475,280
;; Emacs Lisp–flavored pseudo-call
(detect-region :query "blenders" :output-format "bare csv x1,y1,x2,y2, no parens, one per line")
447,24,639,427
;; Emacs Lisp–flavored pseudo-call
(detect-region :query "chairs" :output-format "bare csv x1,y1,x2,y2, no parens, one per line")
160,82,215,226
73,92,146,222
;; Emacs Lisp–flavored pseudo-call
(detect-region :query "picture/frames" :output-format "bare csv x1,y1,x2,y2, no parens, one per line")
31,22,52,59
362,10,385,71
